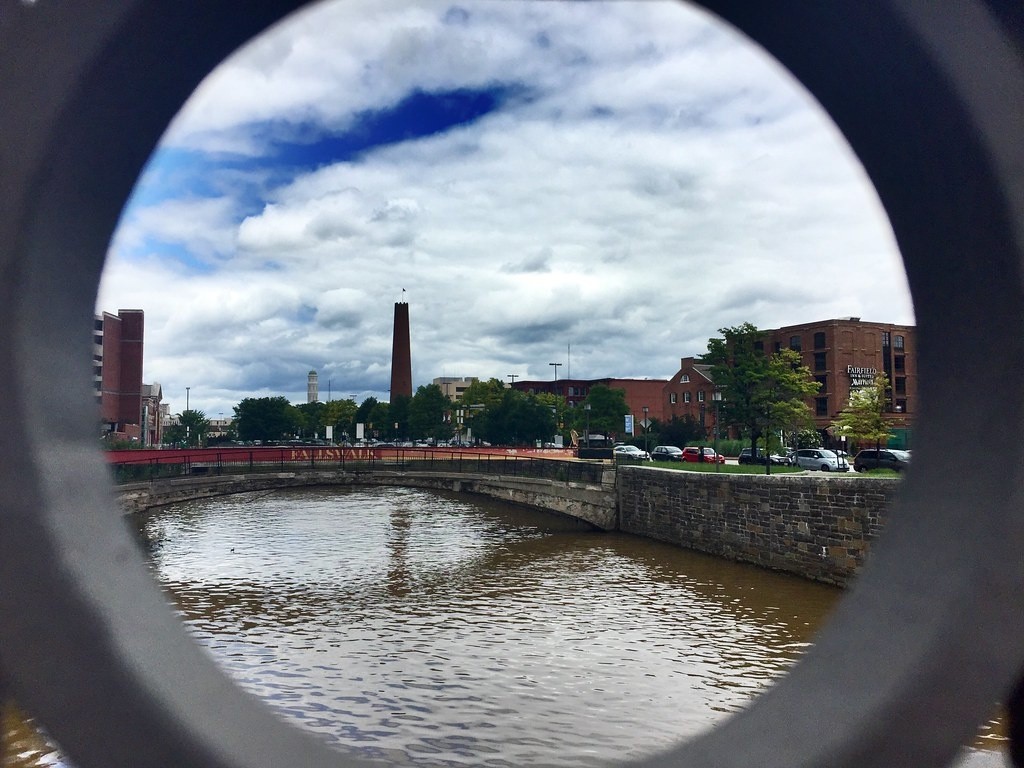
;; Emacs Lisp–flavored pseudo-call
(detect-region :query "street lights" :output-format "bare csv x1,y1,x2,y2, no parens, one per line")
642,406,648,461
584,403,591,447
712,386,722,472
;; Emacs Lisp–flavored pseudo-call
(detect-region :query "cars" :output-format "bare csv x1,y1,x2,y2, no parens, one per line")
230,439,492,448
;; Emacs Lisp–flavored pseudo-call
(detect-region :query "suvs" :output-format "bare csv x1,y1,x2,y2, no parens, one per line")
681,447,725,464
792,449,850,472
854,449,912,473
738,448,792,466
651,446,683,461
614,446,650,461
